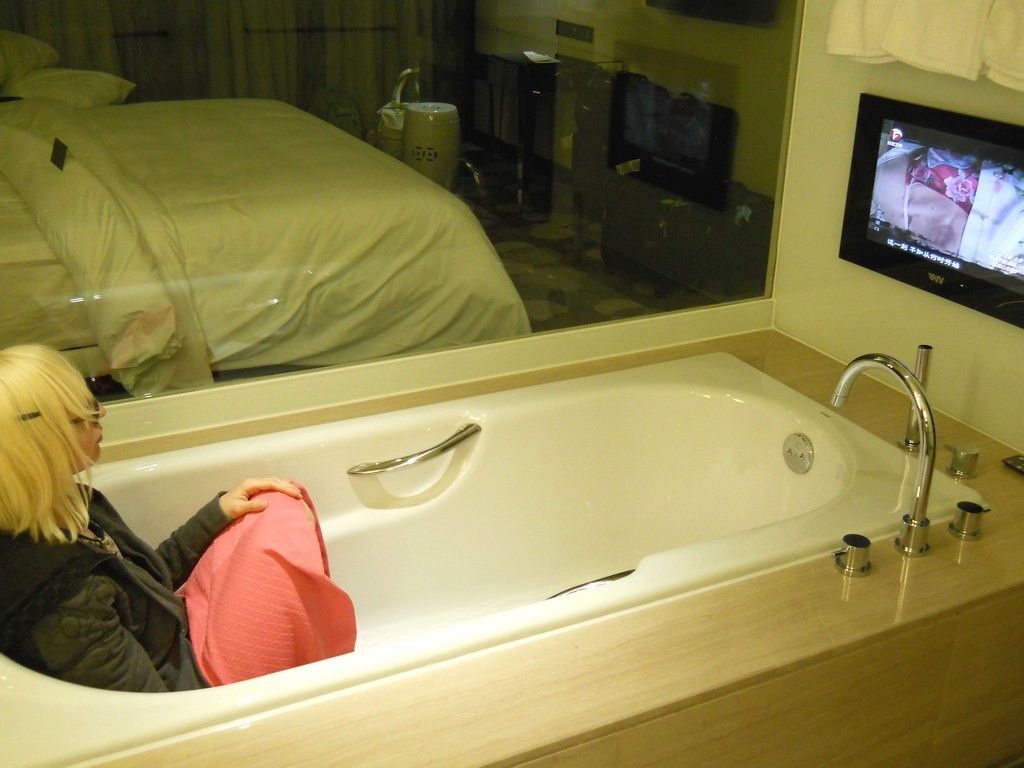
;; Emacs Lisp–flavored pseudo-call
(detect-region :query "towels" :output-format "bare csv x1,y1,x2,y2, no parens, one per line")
178,479,357,687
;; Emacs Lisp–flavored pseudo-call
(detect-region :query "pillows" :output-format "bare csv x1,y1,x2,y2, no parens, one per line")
0,29,61,86
5,68,136,109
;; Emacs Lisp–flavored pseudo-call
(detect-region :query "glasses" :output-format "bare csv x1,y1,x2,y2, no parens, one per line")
70,399,99,426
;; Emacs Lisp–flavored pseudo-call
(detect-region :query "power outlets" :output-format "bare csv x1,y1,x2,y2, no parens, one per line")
556,18,594,43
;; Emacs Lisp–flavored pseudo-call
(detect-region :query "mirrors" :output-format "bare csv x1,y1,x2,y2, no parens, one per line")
0,0,806,406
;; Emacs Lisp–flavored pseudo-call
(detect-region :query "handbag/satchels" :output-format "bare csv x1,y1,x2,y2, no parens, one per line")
378,68,422,160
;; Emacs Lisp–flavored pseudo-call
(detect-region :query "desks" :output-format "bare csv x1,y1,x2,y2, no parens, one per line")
413,22,624,224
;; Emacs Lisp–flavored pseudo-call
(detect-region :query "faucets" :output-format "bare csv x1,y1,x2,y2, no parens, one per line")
830,353,937,558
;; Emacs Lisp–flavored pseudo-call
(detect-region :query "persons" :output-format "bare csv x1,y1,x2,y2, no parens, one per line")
0,344,325,693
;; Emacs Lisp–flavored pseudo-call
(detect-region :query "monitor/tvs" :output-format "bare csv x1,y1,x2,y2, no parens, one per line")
839,93,1024,329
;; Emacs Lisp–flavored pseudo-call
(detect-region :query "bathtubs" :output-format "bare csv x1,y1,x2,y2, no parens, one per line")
1,349,990,768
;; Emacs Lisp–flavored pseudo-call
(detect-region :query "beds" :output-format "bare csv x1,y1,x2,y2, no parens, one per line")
0,98,532,398
